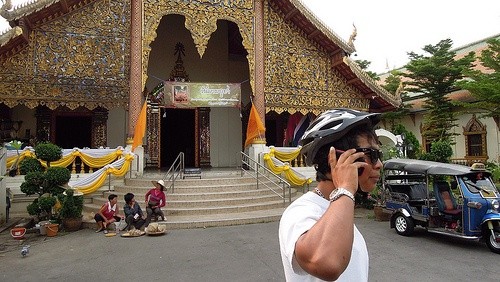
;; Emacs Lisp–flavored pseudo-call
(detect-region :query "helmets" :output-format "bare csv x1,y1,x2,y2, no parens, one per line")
299,109,381,167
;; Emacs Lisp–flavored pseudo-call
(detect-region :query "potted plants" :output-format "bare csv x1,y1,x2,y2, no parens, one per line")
57,188,84,231
19,141,71,224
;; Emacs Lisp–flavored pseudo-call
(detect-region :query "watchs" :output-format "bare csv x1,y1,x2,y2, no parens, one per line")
329,187,355,203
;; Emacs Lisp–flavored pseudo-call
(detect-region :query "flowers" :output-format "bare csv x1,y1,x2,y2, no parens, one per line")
367,185,389,207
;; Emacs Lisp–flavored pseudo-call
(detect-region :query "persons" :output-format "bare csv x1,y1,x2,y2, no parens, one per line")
94,194,125,234
278,108,383,282
146,179,167,227
122,193,146,231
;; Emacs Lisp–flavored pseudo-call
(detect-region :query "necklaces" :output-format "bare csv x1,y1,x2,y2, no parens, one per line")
314,188,324,198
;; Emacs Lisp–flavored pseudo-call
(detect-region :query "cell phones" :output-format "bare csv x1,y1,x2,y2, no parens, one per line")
334,149,365,176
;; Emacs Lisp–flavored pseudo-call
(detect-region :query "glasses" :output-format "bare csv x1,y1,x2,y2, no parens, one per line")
356,148,383,164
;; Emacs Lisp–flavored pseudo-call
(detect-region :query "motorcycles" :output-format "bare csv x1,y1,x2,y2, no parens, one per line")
383,158,500,253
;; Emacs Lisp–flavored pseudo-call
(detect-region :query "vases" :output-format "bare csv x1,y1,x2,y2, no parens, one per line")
373,205,393,221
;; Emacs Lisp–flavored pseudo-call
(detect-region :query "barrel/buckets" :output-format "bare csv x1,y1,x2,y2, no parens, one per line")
10,228,26,239
45,224,59,236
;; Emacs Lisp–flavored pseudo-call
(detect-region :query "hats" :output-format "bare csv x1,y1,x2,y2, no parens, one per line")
152,180,167,191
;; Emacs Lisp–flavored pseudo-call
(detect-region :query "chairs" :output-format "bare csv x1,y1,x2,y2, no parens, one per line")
433,176,462,216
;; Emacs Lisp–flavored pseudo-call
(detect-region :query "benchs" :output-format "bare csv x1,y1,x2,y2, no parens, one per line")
389,182,437,207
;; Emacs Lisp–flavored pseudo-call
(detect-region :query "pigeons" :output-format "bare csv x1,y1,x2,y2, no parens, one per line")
20,244,30,257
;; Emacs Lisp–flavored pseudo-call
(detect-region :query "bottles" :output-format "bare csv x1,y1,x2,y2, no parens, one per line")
116,222,119,233
104,223,108,234
